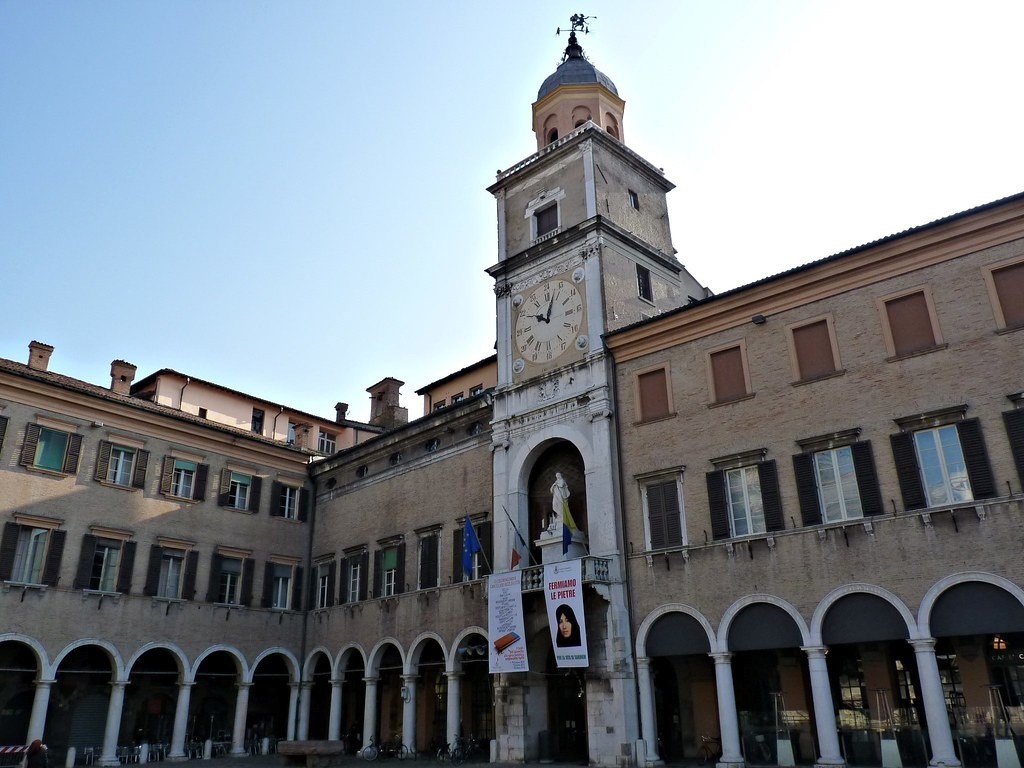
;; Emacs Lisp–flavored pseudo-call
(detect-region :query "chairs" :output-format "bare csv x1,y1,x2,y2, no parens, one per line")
85,736,284,766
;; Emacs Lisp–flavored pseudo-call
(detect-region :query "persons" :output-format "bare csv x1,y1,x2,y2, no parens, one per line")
550,472,570,529
26,739,47,768
556,604,581,648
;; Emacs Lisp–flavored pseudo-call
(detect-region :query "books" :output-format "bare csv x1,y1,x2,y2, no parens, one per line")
494,632,520,651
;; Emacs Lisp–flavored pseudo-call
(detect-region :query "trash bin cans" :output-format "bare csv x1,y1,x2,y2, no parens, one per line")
490,740,496,763
635,738,648,768
261,737,269,755
203,739,212,759
139,743,148,765
65,747,76,768
538,730,554,764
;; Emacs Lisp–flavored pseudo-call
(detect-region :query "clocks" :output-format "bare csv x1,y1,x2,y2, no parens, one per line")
516,279,584,364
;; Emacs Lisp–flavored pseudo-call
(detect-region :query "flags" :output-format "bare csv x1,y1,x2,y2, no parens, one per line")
511,530,530,570
462,513,481,576
561,499,578,554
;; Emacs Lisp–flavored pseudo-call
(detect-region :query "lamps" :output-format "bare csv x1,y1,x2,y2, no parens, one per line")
441,428,454,435
823,645,831,655
576,392,591,407
751,315,766,324
91,421,104,428
577,691,583,698
401,687,408,700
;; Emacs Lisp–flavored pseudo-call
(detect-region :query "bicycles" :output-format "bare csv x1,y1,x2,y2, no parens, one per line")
436,734,485,767
695,736,721,766
361,735,408,762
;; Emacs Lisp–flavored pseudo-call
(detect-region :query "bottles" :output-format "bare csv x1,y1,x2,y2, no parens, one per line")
940,668,1024,737
